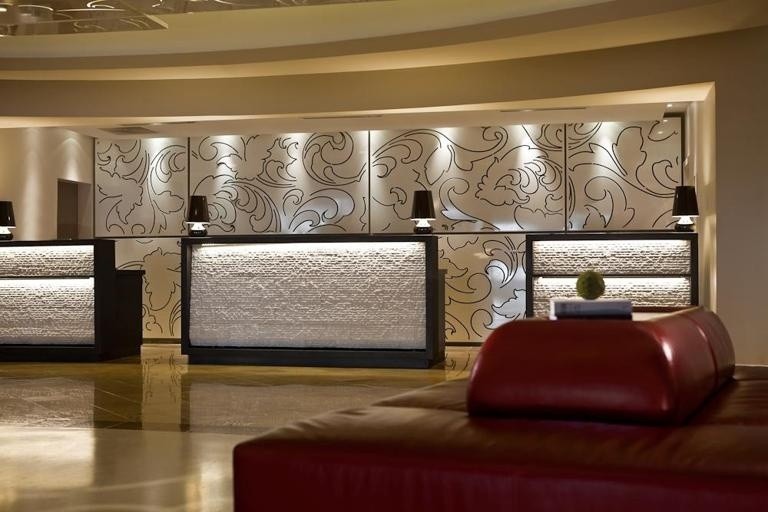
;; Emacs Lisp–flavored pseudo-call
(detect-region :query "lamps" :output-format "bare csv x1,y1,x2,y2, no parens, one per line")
0,200,17,241
411,190,437,234
670,187,700,231
185,195,211,237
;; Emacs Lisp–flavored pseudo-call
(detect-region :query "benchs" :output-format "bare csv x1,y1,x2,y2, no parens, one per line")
230,305,768,512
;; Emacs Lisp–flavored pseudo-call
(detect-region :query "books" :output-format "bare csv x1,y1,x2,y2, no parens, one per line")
549,296,632,319
